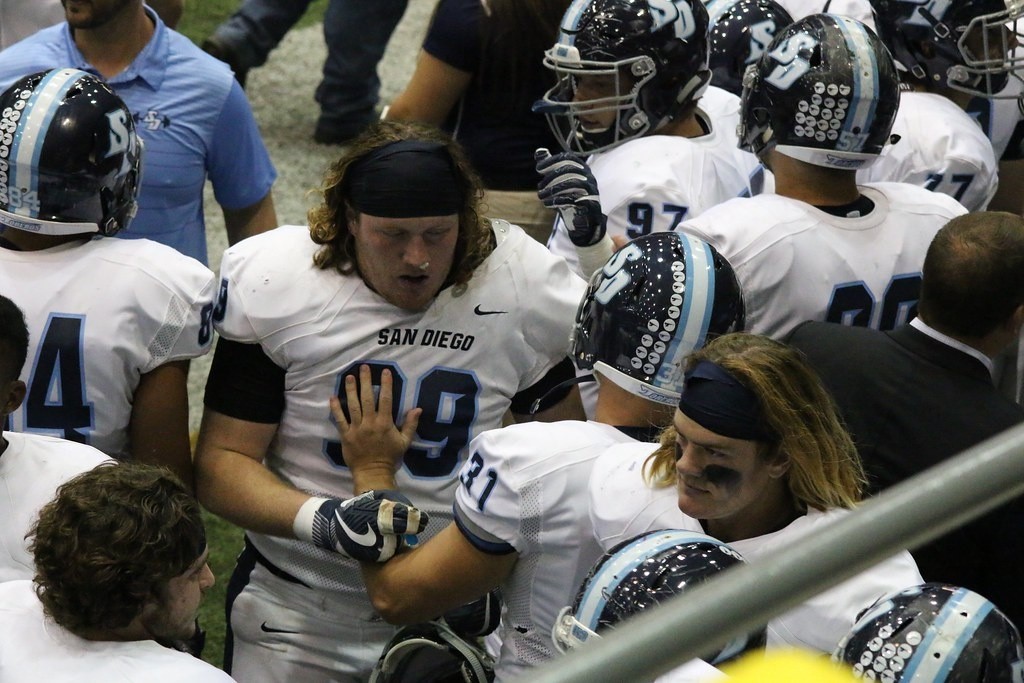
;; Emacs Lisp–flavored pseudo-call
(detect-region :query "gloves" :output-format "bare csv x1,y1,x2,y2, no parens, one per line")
293,490,430,562
431,591,500,638
534,148,608,247
174,617,206,659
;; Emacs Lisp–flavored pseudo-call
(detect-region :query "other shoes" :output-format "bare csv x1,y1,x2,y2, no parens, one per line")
314,114,380,145
201,34,249,90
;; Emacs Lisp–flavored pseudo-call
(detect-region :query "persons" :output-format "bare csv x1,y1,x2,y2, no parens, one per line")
0,0,1024,683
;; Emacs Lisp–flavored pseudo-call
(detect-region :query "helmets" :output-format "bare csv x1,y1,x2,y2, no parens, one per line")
869,0,1024,100
532,0,709,157
572,231,746,407
736,13,900,170
368,621,488,683
831,582,1024,683
0,68,145,238
700,0,794,97
551,528,767,666
